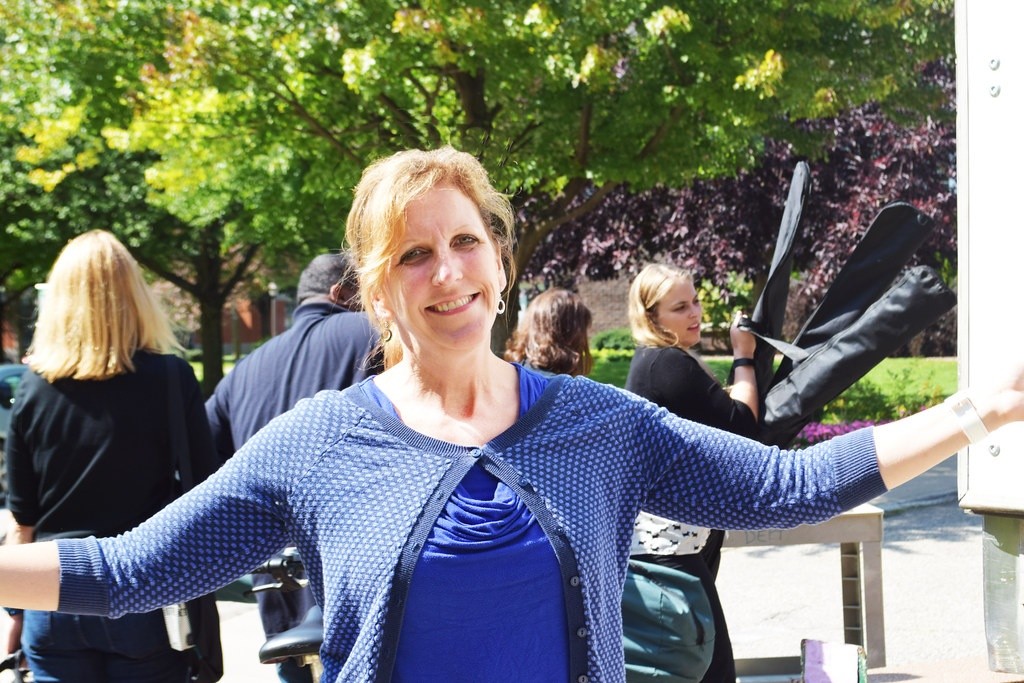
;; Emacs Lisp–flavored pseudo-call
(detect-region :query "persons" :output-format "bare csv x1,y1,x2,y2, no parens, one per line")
0,145,1024,683
4,227,224,683
624,263,759,683
503,286,595,377
205,254,385,683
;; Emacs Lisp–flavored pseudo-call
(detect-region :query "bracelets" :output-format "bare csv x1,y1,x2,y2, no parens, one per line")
943,390,989,443
3,607,21,616
733,358,755,370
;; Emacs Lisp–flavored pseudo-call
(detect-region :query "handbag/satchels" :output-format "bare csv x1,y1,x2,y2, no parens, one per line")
186,592,223,683
620,559,715,683
